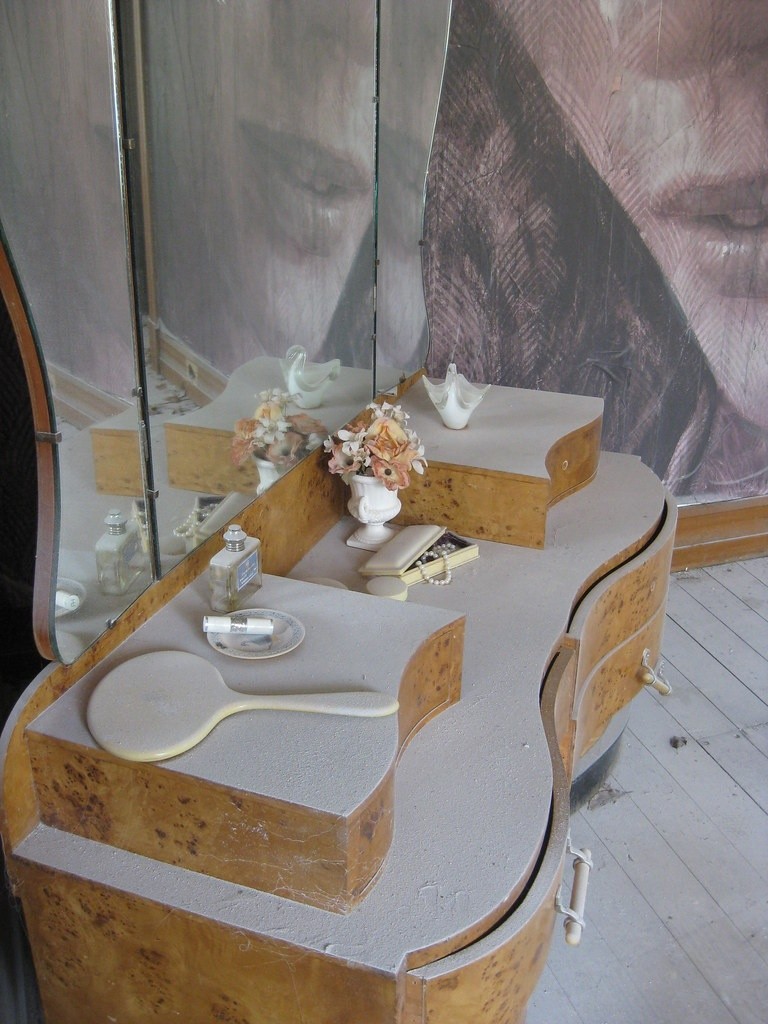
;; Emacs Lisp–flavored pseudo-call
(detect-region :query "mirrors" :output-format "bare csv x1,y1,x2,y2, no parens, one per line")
0,0,454,664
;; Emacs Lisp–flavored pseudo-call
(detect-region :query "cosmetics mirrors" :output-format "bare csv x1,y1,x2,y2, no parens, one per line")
86,647,401,764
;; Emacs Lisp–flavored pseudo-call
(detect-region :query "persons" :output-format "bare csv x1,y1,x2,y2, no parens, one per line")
0,0,446,410
412,0,766,512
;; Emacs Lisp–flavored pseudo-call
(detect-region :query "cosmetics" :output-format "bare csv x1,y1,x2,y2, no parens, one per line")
54,589,80,610
366,577,408,600
202,614,275,636
95,509,146,597
209,524,263,613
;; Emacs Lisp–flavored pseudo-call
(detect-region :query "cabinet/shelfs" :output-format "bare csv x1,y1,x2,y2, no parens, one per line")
24,371,679,1024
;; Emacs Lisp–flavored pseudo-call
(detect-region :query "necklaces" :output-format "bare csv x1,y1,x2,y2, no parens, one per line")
416,543,456,585
173,502,219,538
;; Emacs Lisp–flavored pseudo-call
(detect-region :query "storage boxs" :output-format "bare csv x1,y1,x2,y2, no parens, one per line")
356,522,479,588
